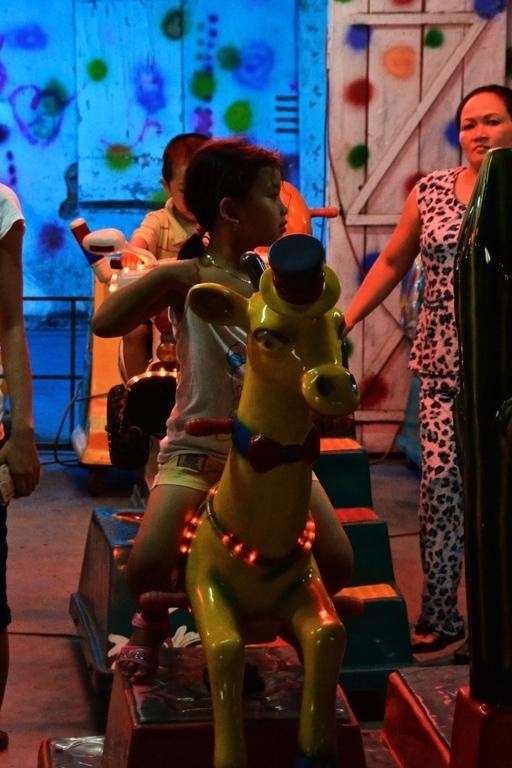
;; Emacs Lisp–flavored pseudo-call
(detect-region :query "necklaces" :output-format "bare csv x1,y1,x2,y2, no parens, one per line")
202,250,260,285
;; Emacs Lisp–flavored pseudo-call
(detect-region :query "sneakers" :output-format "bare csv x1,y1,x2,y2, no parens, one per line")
411,616,464,654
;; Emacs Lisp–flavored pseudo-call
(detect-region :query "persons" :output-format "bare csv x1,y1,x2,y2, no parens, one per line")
91,134,355,605
340,84,512,667
0,177,42,751
119,131,221,385
